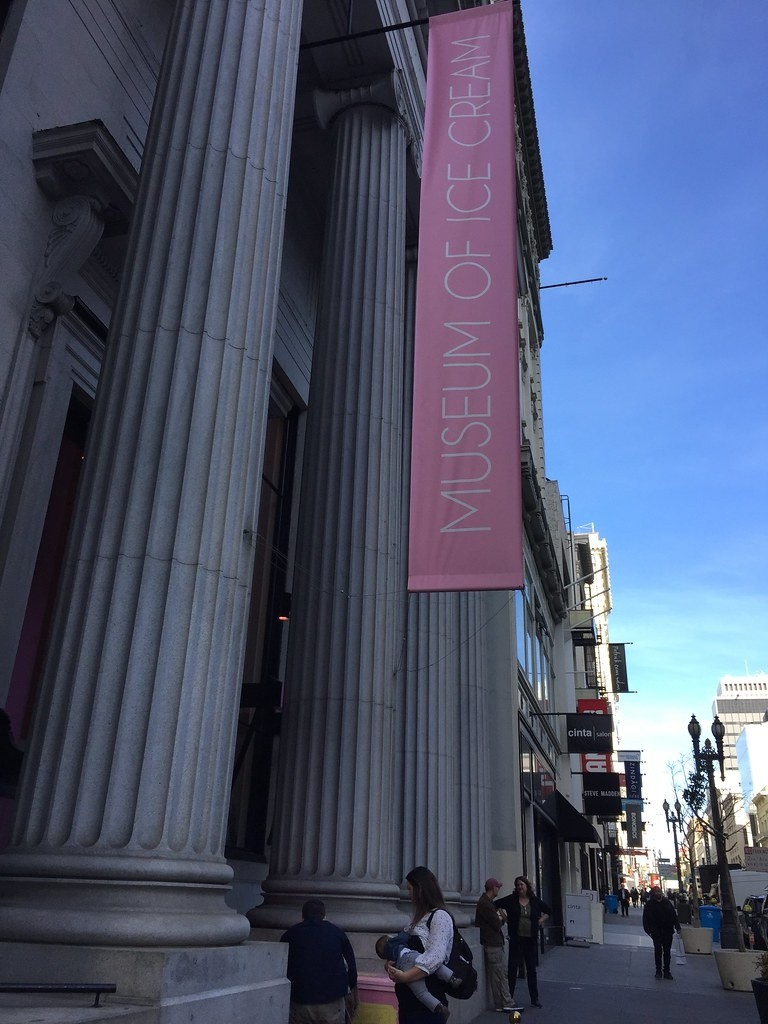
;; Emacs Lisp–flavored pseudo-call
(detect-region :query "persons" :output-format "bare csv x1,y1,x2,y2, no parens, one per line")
643,886,681,979
384,866,454,1024
737,905,756,950
375,925,462,1021
475,878,525,1013
279,899,357,1024
617,883,722,925
493,876,552,1009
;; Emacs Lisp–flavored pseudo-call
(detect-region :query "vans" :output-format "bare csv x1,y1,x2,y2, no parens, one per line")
717,867,768,911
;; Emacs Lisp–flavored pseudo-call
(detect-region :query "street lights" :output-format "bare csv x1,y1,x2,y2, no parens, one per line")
663,799,684,892
689,716,746,950
654,850,662,889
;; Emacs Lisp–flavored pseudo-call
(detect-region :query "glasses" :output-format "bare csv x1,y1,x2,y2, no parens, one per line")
524,906,528,915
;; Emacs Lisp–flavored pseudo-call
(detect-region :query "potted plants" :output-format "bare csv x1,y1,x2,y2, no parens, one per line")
749,952,768,1024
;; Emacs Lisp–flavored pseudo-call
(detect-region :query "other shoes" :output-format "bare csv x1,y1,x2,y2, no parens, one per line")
664,972,673,980
496,1005,525,1012
448,975,463,989
626,915,628,917
530,1002,543,1009
434,1004,450,1024
655,970,662,978
621,915,624,917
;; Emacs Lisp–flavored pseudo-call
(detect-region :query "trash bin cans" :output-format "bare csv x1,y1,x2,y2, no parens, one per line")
606,895,618,914
677,903,692,924
698,906,722,943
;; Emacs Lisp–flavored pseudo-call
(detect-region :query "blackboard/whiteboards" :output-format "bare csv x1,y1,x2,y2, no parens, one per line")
565,894,593,939
581,889,598,902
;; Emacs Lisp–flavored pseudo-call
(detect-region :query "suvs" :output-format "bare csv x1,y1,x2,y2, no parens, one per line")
738,894,768,949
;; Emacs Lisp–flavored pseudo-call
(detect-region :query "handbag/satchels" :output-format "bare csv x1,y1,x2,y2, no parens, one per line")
671,934,687,965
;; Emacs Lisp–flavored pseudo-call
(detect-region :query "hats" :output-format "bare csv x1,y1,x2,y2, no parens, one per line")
485,878,503,887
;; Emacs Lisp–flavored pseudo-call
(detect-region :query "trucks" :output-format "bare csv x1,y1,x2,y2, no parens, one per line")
686,864,743,914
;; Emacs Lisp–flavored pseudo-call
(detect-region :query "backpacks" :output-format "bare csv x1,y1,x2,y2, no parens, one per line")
426,907,478,1000
